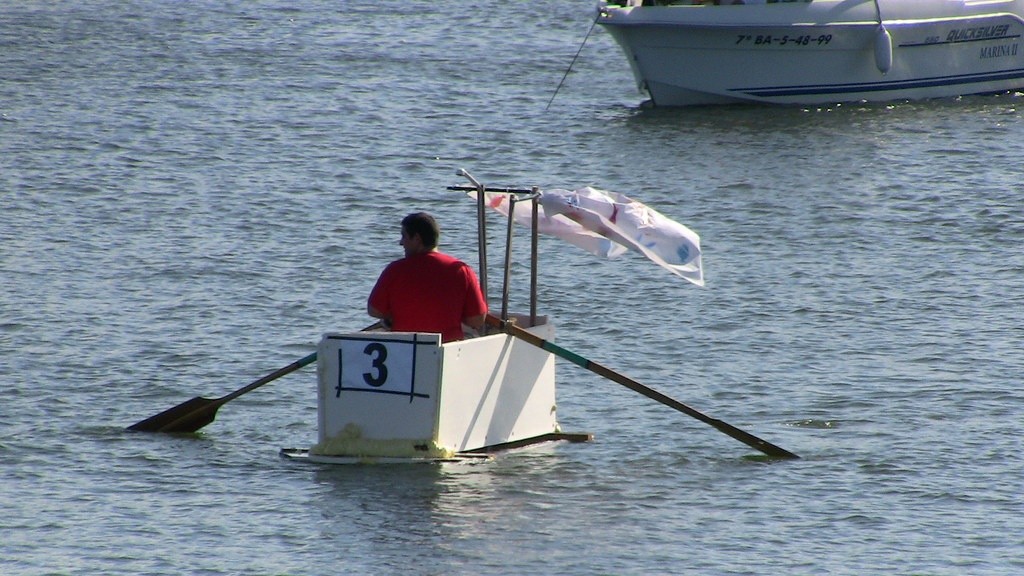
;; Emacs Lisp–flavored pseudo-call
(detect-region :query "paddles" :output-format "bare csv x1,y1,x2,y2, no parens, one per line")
126,318,393,433
485,312,802,460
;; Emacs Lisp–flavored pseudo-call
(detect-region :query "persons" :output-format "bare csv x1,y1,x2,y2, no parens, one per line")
367,213,488,344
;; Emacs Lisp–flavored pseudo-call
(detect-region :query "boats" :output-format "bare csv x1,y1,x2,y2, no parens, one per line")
592,0,1024,112
279,166,556,467
